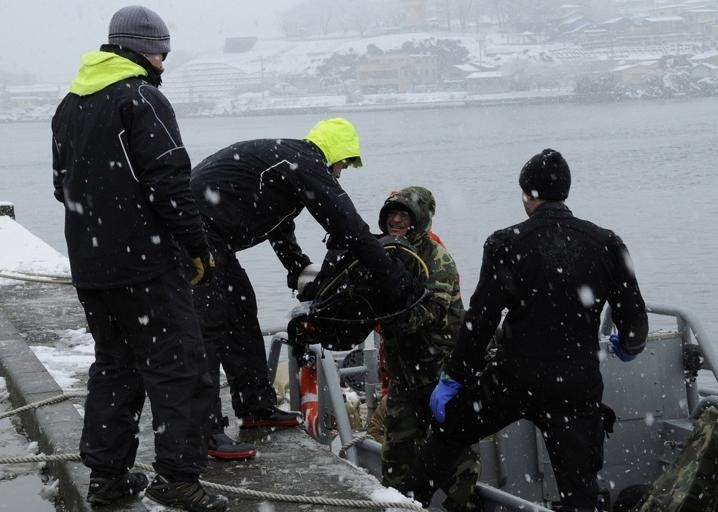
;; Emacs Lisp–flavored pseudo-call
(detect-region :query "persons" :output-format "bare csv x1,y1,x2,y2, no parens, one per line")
301,185,480,510
422,148,649,512
191,117,405,460
52,5,230,512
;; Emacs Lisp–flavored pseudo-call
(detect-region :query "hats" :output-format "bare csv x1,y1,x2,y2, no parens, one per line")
108,5,171,55
519,148,572,201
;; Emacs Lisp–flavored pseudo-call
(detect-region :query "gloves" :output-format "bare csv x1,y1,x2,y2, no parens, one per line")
286,262,314,291
609,333,637,363
429,370,463,425
380,258,414,300
188,250,217,288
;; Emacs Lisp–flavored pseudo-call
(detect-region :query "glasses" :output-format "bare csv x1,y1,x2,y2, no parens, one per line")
386,210,412,219
160,52,168,61
341,160,350,169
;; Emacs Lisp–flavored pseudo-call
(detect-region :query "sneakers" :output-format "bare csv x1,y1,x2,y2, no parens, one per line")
243,405,305,428
144,473,229,512
85,470,149,506
206,432,257,459
